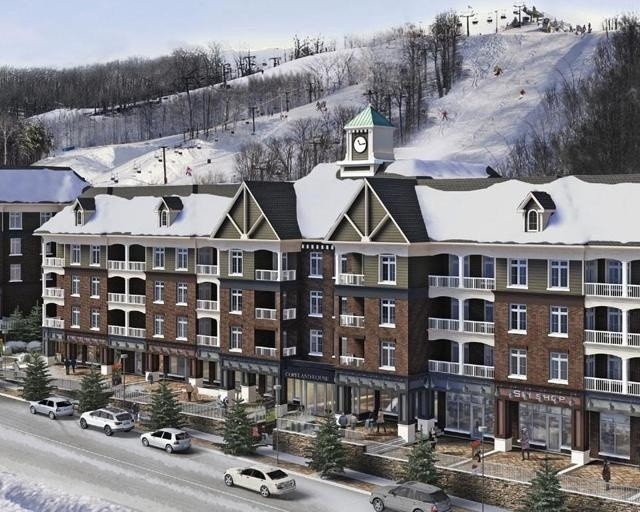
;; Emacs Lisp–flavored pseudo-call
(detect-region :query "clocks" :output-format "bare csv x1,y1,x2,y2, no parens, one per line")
353,135,367,154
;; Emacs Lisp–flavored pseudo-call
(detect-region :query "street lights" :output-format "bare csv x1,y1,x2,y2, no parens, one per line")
273,385,282,464
121,354,128,409
2,330,8,381
478,426,488,512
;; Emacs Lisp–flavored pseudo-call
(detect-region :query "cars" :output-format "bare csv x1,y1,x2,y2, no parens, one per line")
140,428,191,453
370,481,451,512
30,396,74,420
223,466,296,497
80,407,135,436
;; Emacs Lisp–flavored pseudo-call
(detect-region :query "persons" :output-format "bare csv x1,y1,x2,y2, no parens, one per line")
338,412,348,429
365,413,376,434
517,428,530,461
351,413,358,430
186,382,194,401
192,385,198,401
70,358,76,373
429,427,438,450
601,460,611,490
64,359,71,375
376,407,386,432
